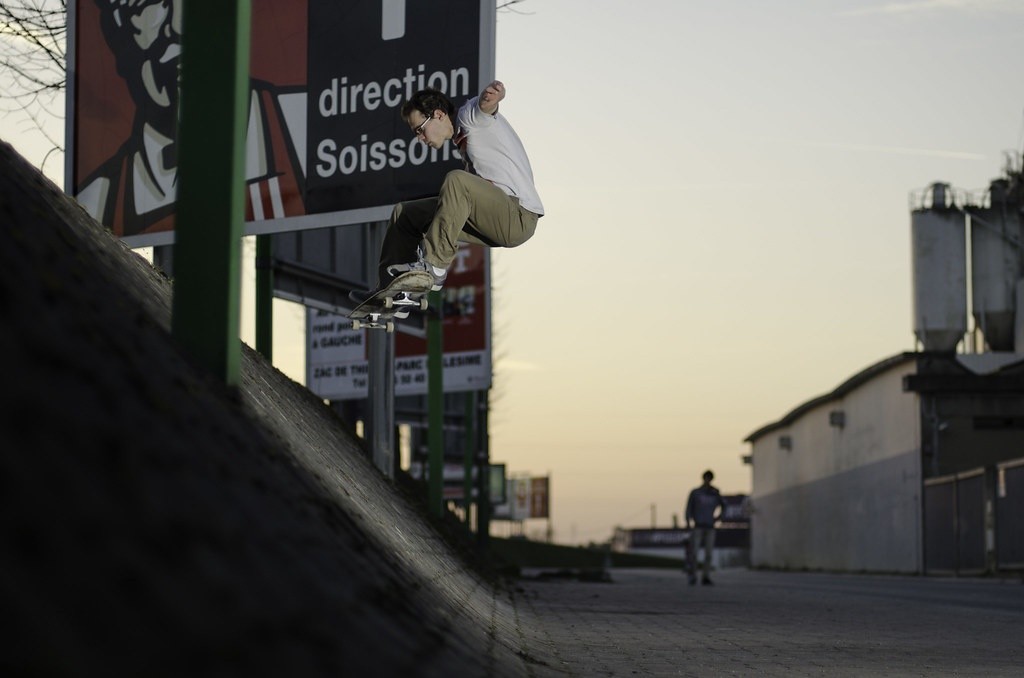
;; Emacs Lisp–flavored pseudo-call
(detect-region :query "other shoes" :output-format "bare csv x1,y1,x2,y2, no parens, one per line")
702,577,713,586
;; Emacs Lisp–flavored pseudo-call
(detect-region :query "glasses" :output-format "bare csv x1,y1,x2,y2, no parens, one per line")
412,116,432,137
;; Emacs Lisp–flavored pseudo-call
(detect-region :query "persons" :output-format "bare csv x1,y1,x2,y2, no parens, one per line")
348,81,543,318
685,470,726,586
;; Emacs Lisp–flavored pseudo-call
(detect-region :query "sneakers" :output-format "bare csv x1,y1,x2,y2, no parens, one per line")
385,245,448,292
349,282,410,318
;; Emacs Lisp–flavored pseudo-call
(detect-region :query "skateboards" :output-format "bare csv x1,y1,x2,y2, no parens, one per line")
344,271,433,334
